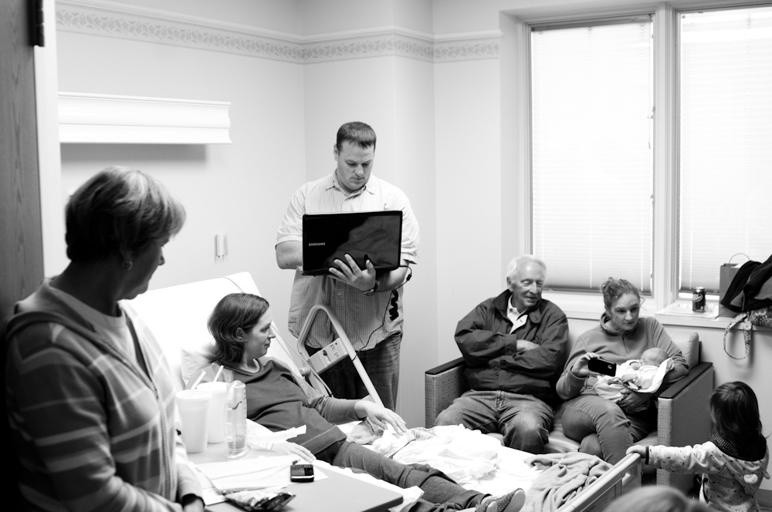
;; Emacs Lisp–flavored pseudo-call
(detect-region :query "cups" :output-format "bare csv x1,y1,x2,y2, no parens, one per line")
175,379,227,458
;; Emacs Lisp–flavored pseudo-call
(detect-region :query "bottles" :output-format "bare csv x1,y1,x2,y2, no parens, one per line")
227,380,247,455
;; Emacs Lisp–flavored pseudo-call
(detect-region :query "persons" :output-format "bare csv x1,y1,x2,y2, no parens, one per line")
624,379,771,512
599,483,715,512
272,120,422,414
430,252,572,456
1,164,225,512
556,276,692,473
590,346,672,401
196,290,528,511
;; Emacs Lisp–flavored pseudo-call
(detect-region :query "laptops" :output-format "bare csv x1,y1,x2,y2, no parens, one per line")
296,210,403,276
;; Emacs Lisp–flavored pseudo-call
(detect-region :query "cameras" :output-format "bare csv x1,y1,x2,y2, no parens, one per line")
587,358,617,377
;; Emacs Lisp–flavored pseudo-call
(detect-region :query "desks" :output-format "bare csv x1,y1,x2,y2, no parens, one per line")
172,437,404,512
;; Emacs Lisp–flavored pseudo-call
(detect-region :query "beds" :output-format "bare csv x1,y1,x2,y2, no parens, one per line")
118,272,643,512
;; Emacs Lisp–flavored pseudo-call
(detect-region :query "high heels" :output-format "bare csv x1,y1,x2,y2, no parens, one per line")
718,254,772,359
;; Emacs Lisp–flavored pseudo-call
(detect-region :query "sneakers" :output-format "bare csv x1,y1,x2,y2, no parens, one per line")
474,488,526,512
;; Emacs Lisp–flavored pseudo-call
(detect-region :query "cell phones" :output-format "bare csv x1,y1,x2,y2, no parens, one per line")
290,464,314,482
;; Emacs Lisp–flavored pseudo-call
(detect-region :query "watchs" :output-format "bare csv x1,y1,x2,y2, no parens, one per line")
360,283,379,296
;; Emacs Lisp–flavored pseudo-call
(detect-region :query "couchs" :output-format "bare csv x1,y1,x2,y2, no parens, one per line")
425,330,714,495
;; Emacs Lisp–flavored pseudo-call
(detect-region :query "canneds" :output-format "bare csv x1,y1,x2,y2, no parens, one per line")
692,288,705,313
